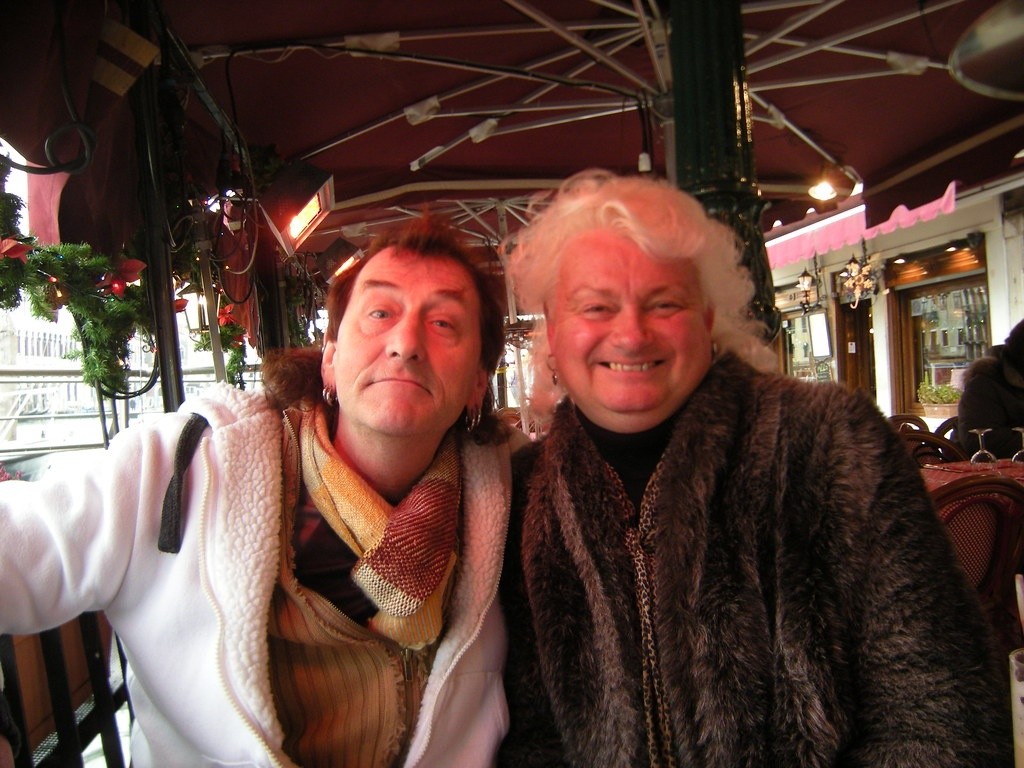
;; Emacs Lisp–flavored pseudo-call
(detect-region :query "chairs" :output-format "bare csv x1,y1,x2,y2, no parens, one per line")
886,415,968,465
931,475,1024,653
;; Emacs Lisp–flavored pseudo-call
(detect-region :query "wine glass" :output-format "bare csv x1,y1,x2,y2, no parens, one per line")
968,429,996,464
1011,428,1024,462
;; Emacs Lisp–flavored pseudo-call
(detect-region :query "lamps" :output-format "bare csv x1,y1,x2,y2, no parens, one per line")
838,236,877,309
316,237,365,286
252,156,337,258
797,252,821,314
808,154,839,202
944,242,959,253
892,253,908,265
217,148,269,208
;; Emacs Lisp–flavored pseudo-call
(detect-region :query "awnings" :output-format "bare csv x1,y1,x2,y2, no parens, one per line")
762,159,1024,268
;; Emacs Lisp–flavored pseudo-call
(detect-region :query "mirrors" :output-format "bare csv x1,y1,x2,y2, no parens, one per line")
806,308,833,360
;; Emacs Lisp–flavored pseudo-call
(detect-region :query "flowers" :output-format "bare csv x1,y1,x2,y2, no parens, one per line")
95,254,147,301
217,304,238,327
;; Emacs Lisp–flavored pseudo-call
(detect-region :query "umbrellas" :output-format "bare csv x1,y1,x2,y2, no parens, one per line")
2,1,1024,438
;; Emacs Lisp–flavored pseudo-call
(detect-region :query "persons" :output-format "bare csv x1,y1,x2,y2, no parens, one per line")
497,171,1008,768
0,221,550,768
943,314,1024,466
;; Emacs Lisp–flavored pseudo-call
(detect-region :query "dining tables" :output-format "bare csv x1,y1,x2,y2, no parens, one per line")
916,458,1024,499
